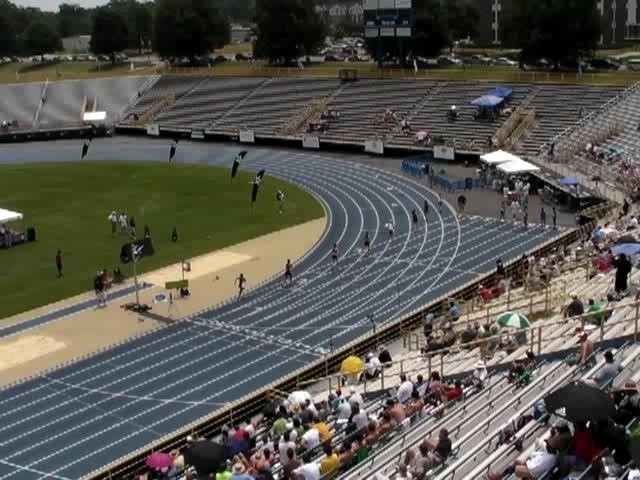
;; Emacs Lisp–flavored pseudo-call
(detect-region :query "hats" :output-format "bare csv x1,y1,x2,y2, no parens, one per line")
604,351,613,357
232,463,246,473
617,378,639,392
578,333,588,340
526,350,534,358
544,436,560,449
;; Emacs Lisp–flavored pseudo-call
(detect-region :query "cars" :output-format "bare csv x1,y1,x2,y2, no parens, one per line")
319,35,369,62
1,53,131,65
406,54,639,72
200,52,250,63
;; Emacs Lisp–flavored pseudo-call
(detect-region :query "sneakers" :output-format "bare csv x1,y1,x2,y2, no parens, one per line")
487,470,497,479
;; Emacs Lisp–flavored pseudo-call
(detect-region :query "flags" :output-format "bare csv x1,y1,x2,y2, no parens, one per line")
183,260,190,271
169,136,180,161
252,169,265,202
231,150,247,178
120,237,155,262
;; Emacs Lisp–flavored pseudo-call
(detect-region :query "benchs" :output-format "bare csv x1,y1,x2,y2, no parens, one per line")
118,77,640,479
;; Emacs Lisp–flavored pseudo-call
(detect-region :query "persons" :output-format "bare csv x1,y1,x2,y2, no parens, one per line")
473,107,639,229
56,249,64,279
95,268,110,307
107,211,137,240
411,192,443,234
4,226,12,249
423,162,434,177
234,273,246,302
180,287,190,298
307,111,341,134
277,190,284,209
421,214,640,337
285,259,294,282
465,159,468,168
143,223,151,237
139,330,640,480
331,219,393,267
382,106,461,147
458,195,467,214
